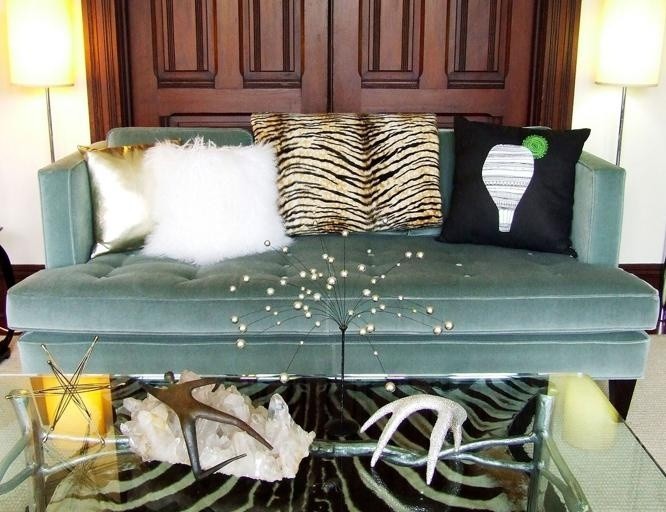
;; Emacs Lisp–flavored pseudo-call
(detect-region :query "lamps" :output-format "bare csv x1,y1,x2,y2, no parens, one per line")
0,0,78,164
589,0,666,167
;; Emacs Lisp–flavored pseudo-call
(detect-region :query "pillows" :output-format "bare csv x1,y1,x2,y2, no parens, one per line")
434,116,591,258
77,139,182,259
251,112,443,236
141,133,295,265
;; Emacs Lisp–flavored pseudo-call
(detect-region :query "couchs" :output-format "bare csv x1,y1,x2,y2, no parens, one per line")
5,126,663,420
